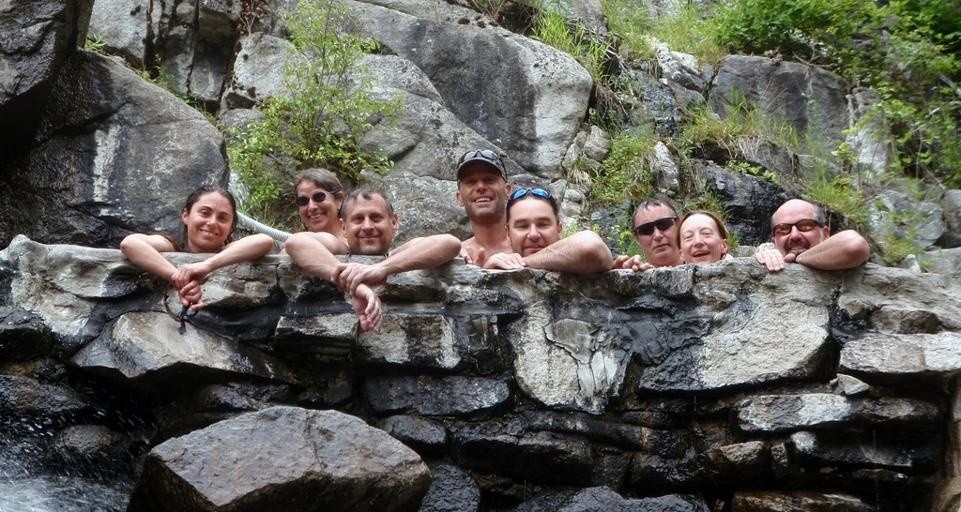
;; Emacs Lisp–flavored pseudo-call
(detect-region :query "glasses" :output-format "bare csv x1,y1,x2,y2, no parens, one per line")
297,191,334,205
633,216,677,235
773,219,821,235
511,187,552,200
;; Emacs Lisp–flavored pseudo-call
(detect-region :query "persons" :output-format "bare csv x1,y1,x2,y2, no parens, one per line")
119,147,872,333
